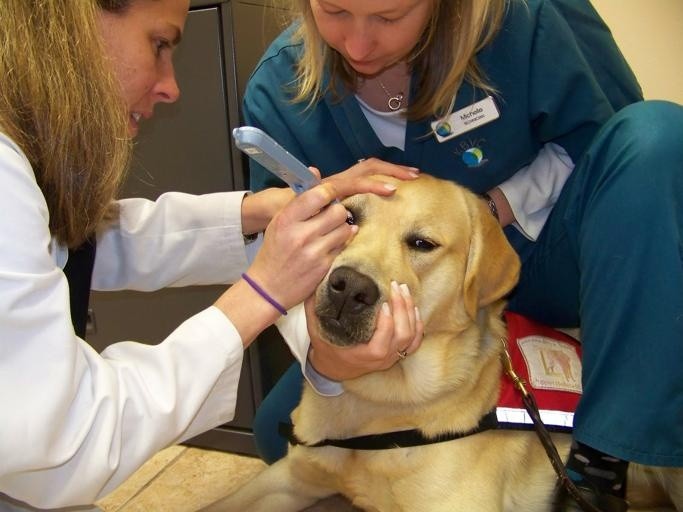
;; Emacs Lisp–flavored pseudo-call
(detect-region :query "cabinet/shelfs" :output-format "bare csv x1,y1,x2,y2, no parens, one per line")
79,1,312,464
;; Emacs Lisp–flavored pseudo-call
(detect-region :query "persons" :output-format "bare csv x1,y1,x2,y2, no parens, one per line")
235,0,680,509
0,0,420,512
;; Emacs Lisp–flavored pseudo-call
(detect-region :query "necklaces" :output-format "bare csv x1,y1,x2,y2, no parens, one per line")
370,68,406,111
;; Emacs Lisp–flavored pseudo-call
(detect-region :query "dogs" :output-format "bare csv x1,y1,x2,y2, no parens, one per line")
195,172,683,512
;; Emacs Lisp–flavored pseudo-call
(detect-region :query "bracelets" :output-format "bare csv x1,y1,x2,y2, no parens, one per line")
240,271,289,316
482,192,499,223
